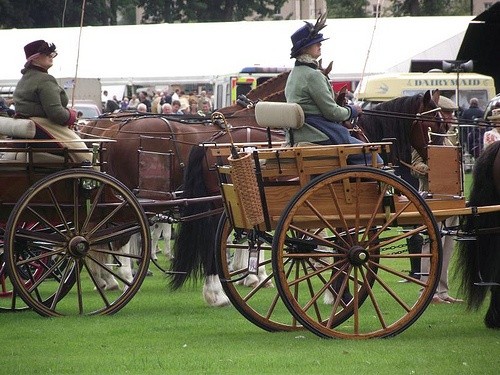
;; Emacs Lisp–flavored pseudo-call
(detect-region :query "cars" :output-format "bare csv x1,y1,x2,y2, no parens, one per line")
63,100,103,127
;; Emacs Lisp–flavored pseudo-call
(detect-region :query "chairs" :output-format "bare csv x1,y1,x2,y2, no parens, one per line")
1,115,70,163
254,101,351,182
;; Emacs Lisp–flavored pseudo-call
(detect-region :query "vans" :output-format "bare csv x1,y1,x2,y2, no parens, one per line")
351,72,495,115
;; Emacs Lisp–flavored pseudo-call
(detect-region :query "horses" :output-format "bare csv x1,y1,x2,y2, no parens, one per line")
448,140,500,330
80,59,351,291
167,88,455,306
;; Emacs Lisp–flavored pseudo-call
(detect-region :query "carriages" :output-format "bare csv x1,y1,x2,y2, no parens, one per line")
0,56,335,321
198,106,500,346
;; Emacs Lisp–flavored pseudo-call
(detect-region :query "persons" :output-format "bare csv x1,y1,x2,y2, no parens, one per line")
285,22,398,195
99,84,217,118
416,94,464,307
458,96,486,159
396,227,422,283
12,40,98,168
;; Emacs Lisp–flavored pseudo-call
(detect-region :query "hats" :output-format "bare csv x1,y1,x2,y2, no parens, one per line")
290,10,330,59
24,40,57,61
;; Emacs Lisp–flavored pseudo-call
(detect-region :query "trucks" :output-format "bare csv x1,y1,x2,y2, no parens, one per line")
210,66,297,115
